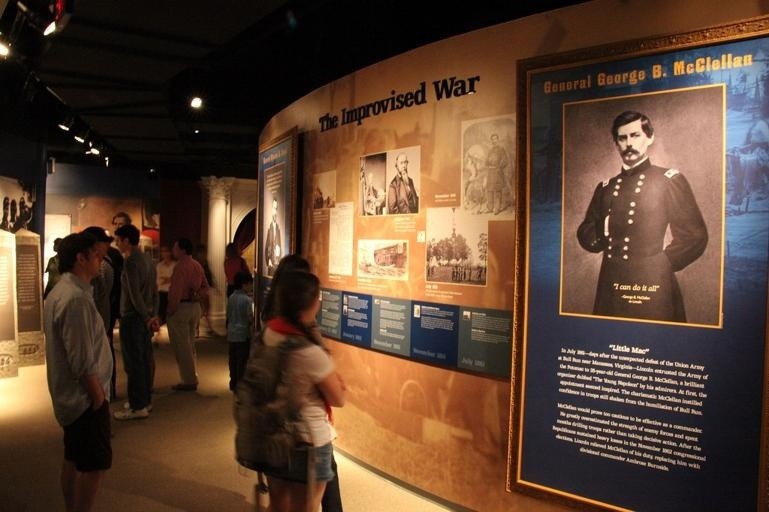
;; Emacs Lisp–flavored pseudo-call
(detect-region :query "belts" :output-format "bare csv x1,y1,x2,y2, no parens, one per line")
180,297,200,303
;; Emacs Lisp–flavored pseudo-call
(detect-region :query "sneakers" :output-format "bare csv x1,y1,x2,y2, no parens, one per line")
172,382,197,392
113,400,153,420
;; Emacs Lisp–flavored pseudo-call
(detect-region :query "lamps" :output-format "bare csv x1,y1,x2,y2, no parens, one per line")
47,87,115,168
29,10,57,36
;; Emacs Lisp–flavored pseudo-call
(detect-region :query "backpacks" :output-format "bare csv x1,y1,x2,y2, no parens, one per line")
233,323,316,478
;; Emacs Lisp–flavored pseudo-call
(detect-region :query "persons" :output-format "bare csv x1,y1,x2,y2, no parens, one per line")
261,252,346,512
226,268,256,394
577,109,709,326
250,265,349,512
387,152,419,216
223,243,253,337
43,231,115,510
45,223,209,422
15,186,37,233
738,109,769,191
0,196,13,233
264,196,283,276
483,133,510,216
108,213,154,260
